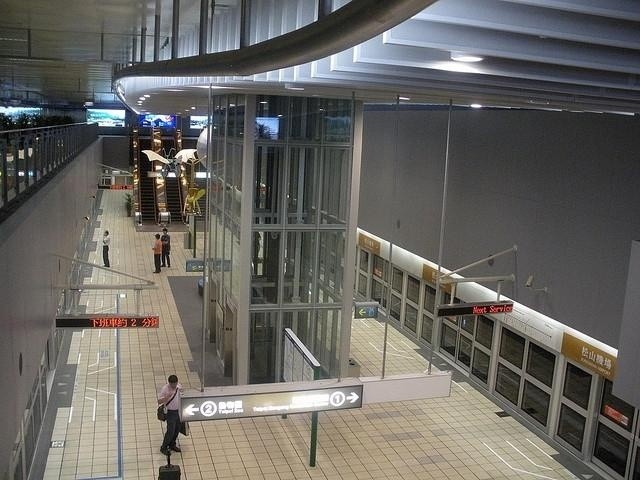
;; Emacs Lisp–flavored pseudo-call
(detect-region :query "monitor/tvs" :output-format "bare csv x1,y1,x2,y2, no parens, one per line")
256,116,280,137
188,114,209,129
85,108,126,127
139,113,177,129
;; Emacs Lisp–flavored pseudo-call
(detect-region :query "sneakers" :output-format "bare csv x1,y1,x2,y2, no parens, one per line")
160,446,171,455
169,445,181,452
104,265,110,267
153,264,170,273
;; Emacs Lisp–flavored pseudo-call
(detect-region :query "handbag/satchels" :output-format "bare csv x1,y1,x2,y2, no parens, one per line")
157,404,167,422
180,422,191,436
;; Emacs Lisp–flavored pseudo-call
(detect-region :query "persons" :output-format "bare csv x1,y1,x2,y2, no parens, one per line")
161,228,172,267
102,229,110,268
156,374,184,456
151,233,163,272
